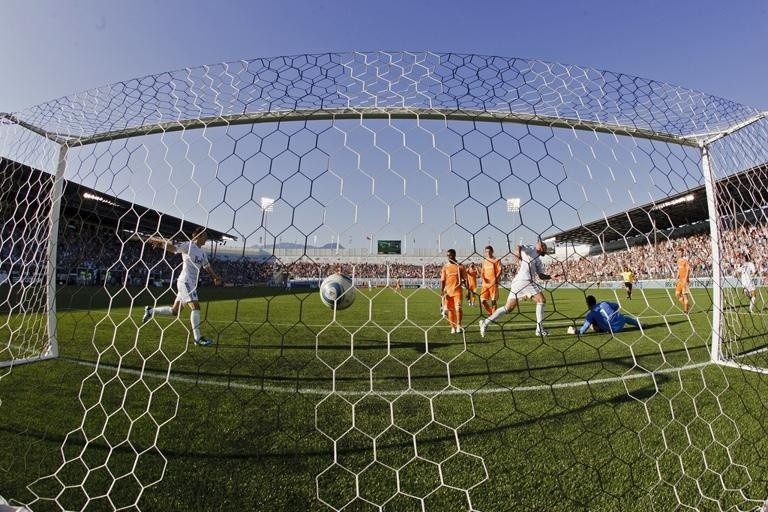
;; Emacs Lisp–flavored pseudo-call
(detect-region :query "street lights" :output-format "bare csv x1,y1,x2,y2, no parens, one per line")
260,195,275,249
505,197,523,258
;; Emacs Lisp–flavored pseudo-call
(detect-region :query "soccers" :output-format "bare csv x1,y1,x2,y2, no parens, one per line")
319,274,354,308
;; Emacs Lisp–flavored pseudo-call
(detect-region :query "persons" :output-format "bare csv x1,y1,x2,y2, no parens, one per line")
613,267,637,299
463,262,481,308
675,248,691,314
482,245,502,320
478,241,561,337
567,294,647,334
142,225,222,347
442,249,473,334
733,254,757,312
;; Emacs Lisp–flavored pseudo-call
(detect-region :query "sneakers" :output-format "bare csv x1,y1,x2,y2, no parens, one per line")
142,305,151,323
442,302,475,334
193,336,212,347
478,318,488,338
535,328,553,336
627,296,631,300
683,305,691,315
